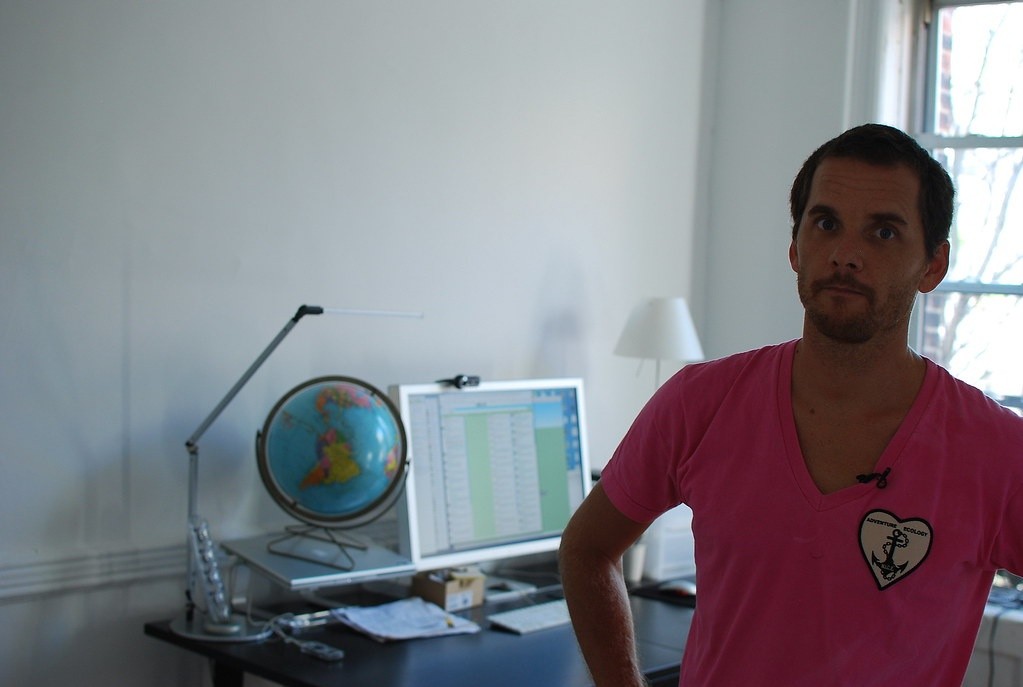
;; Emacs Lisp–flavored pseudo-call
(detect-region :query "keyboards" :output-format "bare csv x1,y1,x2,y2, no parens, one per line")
484,597,572,636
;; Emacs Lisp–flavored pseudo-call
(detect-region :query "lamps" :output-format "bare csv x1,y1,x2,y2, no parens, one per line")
611,293,709,401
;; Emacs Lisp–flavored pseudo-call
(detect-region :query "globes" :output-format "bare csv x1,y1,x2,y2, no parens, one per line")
254,373,411,574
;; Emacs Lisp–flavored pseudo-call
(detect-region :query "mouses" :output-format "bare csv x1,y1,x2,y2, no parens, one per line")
659,579,697,599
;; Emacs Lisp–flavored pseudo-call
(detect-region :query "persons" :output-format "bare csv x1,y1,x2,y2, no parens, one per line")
557,122,1023,687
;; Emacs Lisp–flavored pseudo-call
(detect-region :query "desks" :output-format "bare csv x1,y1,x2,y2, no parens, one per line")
142,558,707,687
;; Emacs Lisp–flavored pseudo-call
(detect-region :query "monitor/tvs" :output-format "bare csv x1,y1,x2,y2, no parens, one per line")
386,376,591,572
